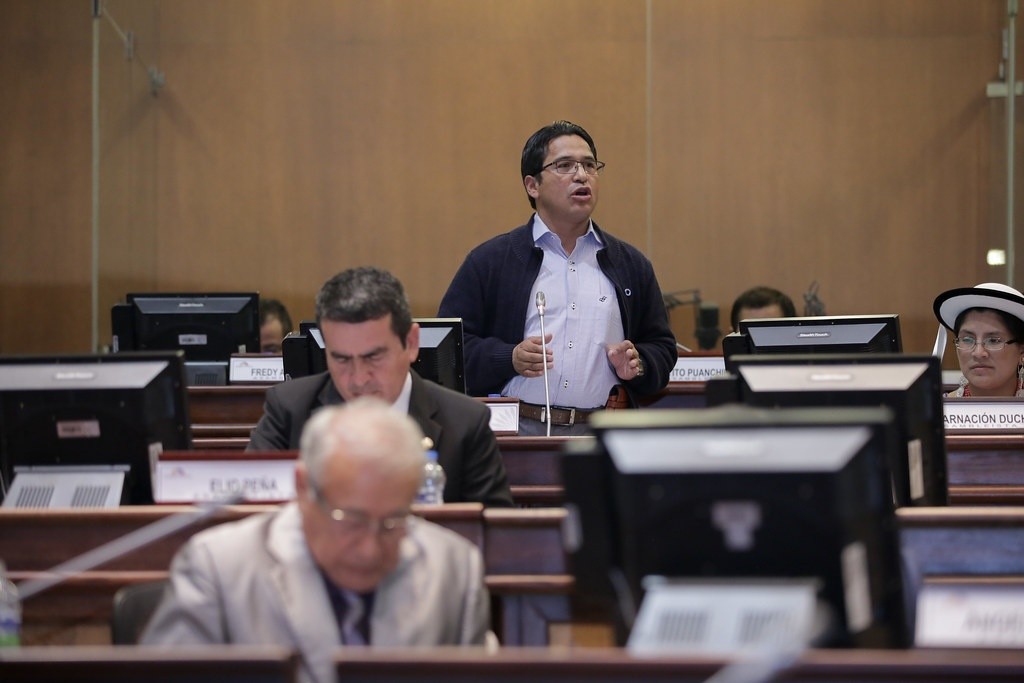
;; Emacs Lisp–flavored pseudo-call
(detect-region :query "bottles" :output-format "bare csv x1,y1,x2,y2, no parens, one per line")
0,560,22,648
415,451,446,505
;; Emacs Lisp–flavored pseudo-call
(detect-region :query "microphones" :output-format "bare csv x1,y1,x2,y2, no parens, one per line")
17,489,245,602
698,302,720,350
536,291,551,436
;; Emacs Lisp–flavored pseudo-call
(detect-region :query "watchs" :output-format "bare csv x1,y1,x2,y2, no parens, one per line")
638,358,645,376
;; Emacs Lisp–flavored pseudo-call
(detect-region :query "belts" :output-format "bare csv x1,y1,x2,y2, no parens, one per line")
519,401,604,427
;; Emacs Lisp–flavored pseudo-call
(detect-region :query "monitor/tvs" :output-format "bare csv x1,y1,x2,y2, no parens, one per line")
112,291,261,362
282,318,468,395
706,354,946,505
0,349,192,506
723,314,902,357
560,406,915,648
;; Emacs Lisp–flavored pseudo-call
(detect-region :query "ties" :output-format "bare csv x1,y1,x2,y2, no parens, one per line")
336,587,369,647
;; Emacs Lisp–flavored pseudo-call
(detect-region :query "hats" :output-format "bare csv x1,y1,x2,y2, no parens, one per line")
933,282,1024,332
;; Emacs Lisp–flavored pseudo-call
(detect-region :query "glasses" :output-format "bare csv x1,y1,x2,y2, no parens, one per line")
951,332,1024,351
305,473,416,540
532,160,606,177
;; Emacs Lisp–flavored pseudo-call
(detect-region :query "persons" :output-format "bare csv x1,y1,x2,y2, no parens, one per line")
257,300,293,355
438,120,679,437
730,287,798,332
933,283,1024,397
242,265,516,510
137,395,503,683
